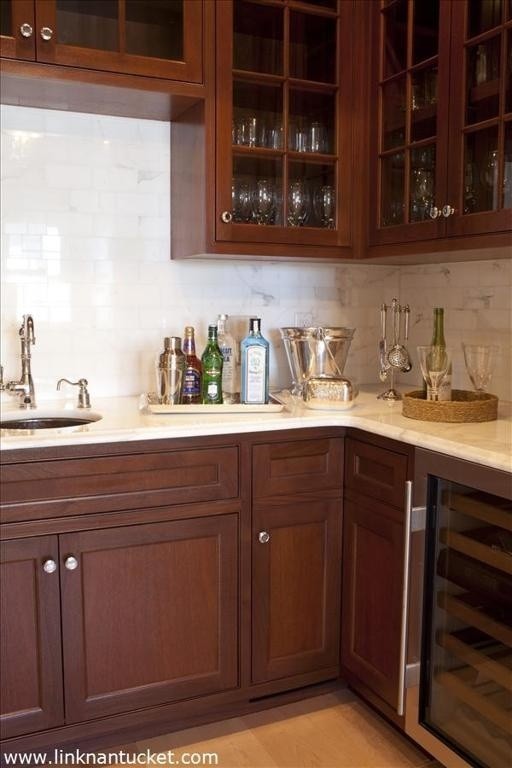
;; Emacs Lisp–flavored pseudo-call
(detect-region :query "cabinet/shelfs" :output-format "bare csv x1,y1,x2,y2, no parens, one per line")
0,0,206,122
170,0,358,263
249,427,348,699
343,428,421,733
0,433,249,767
357,0,512,267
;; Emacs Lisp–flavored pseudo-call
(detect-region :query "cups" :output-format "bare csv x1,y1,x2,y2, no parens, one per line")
399,42,497,113
229,116,330,154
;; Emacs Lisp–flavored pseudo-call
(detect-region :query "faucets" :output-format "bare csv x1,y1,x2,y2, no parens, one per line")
21,312,35,379
57,379,92,409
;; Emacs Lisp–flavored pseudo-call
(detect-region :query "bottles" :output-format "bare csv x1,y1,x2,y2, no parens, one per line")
424,306,452,401
199,325,224,406
154,335,186,406
182,324,203,406
216,314,240,404
241,317,269,405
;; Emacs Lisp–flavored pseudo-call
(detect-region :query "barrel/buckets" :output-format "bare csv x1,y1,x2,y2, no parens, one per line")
280,326,356,396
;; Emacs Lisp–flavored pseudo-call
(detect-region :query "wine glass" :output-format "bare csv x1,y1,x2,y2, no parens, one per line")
462,341,501,403
231,174,333,228
383,145,512,224
415,344,453,401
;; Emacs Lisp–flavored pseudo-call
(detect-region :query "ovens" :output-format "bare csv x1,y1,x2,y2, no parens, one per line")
393,445,512,768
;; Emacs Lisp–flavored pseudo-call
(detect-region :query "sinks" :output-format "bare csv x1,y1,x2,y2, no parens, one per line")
0,408,103,430
